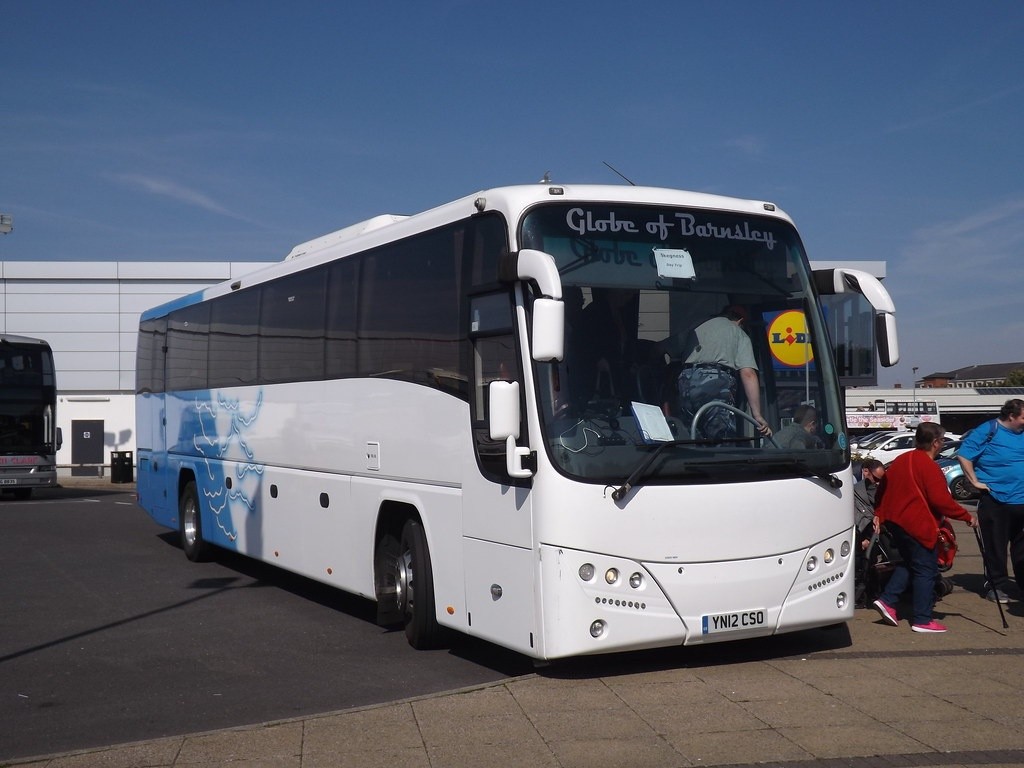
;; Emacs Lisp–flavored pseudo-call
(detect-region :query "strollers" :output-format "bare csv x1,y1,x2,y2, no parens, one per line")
853,489,961,610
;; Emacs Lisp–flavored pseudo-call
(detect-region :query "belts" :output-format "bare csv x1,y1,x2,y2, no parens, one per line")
684,363,734,376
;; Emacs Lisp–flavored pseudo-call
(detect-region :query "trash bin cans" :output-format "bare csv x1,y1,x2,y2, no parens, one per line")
110,451,133,485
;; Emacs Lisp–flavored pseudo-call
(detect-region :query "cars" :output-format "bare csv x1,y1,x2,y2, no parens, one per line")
935,448,986,501
848,428,962,465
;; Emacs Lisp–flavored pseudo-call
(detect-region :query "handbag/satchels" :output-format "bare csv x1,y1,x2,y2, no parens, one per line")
936,519,960,566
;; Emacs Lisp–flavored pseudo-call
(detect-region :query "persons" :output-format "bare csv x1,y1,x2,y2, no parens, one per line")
957,399,1024,603
676,304,772,447
759,405,830,449
852,458,886,604
871,421,979,633
467,345,529,455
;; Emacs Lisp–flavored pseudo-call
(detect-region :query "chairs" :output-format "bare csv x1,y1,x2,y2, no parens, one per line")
538,349,628,427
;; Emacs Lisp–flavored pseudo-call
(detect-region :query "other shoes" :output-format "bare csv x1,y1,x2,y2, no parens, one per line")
986,589,1008,603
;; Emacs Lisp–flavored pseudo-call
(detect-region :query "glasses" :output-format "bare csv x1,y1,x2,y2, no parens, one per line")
931,437,946,445
869,470,880,482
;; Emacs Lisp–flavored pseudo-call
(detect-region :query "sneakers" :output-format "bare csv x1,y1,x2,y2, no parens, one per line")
873,599,899,626
911,620,947,633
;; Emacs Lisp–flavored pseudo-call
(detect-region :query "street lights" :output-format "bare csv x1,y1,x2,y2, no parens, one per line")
911,366,919,418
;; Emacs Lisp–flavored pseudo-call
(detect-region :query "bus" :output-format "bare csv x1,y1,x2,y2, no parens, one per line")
134,172,902,665
845,397,942,431
0,333,64,500
